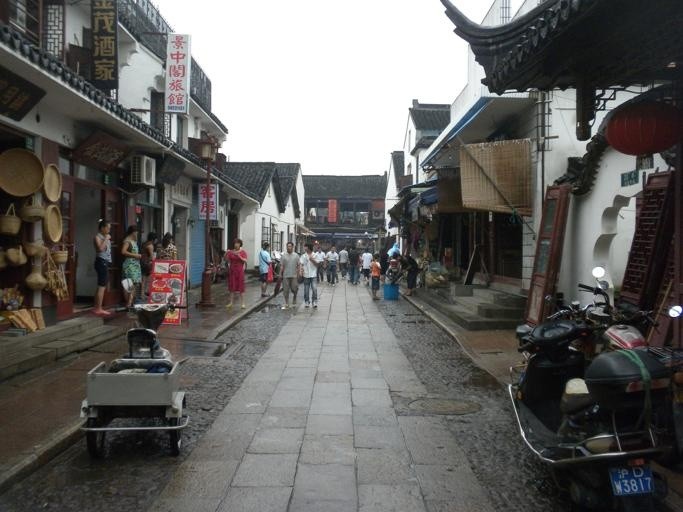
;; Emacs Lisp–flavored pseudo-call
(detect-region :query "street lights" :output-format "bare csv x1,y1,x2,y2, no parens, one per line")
193,137,218,308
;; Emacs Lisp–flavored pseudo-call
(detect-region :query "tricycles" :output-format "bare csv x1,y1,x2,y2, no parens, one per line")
77,300,191,459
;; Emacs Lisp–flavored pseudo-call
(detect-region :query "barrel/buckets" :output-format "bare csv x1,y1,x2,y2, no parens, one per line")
384,283,399,300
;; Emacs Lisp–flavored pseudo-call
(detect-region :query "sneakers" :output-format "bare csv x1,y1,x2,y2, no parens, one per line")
304,300,309,307
312,301,317,308
281,303,289,310
292,302,296,307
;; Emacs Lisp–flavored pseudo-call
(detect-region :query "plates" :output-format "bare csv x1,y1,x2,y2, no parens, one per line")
151,264,182,302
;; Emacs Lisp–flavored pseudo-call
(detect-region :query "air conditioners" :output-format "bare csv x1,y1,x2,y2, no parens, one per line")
209,205,226,232
372,209,384,220
129,149,156,191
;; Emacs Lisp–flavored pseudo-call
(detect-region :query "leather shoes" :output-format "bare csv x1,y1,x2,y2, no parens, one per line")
225,304,231,308
241,304,245,309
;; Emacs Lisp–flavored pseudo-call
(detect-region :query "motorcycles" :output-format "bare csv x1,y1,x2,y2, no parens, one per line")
387,254,399,284
572,266,682,472
501,315,671,512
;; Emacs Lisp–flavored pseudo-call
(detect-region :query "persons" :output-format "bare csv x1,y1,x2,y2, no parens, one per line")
139,232,158,299
224,238,248,309
120,225,143,310
93,219,113,317
258,240,419,311
155,233,178,261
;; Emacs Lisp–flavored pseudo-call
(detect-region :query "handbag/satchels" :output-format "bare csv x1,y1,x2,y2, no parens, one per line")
267,263,272,283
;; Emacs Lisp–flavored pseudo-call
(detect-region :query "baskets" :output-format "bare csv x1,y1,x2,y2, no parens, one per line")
0,148,68,306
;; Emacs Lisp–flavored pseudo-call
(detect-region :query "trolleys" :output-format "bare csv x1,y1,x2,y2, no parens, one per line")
346,264,362,284
